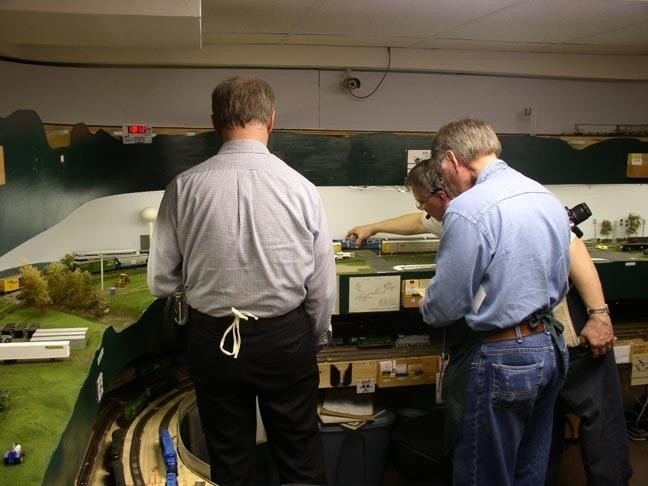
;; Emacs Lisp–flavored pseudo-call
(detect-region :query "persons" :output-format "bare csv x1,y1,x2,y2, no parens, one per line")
146,76,337,486
346,160,636,486
418,119,570,485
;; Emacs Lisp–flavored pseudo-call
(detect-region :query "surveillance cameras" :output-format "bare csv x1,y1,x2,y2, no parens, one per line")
343,77,361,90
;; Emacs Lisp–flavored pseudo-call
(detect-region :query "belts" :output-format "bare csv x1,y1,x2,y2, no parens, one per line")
474,314,547,342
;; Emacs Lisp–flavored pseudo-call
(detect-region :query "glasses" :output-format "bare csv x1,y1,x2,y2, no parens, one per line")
415,193,436,210
435,155,448,189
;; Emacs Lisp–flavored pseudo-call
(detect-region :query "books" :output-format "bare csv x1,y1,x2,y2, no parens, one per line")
316,403,367,425
320,392,378,423
340,408,386,431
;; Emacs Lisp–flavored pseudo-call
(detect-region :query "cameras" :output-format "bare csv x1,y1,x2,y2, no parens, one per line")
563,199,593,238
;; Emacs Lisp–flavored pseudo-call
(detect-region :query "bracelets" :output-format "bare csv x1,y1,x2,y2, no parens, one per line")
586,303,610,314
